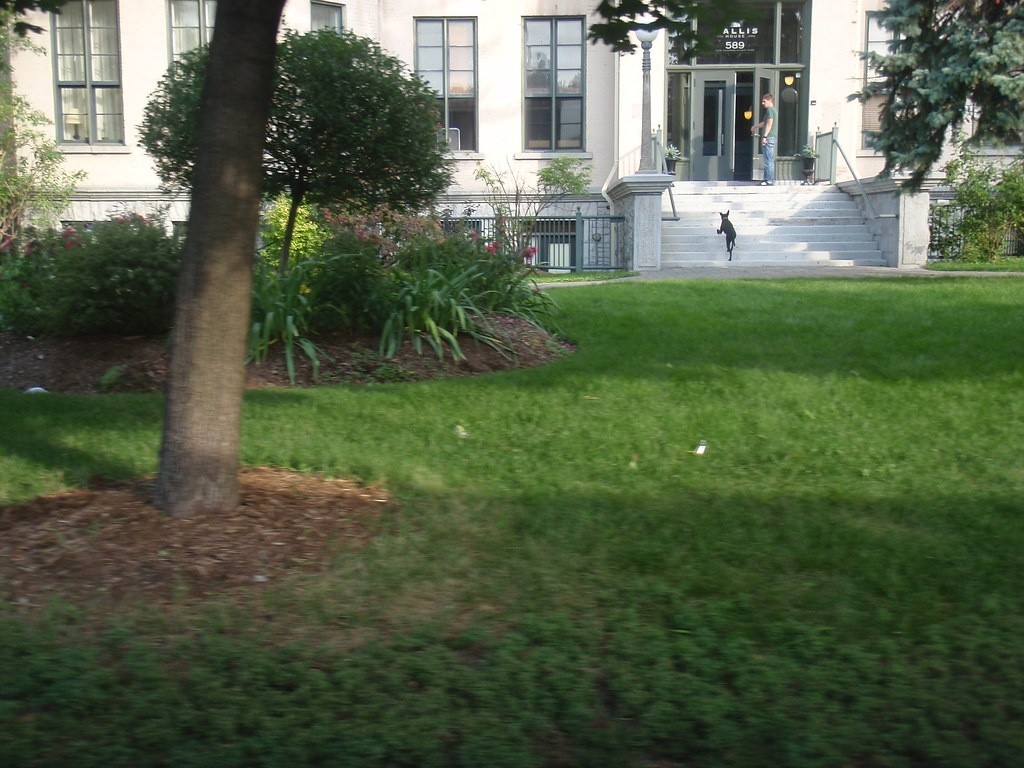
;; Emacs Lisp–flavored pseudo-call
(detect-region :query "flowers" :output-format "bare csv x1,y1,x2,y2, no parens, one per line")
465,229,575,355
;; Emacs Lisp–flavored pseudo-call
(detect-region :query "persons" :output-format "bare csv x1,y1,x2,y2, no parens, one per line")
751,93,777,186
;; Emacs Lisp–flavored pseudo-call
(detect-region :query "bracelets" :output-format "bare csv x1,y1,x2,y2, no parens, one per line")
763,136,767,138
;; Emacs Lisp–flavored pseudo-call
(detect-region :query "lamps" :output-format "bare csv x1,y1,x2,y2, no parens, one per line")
65,107,82,140
783,71,794,85
742,99,754,120
782,88,798,103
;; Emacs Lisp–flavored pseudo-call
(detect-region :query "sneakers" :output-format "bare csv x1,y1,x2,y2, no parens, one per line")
757,180,773,186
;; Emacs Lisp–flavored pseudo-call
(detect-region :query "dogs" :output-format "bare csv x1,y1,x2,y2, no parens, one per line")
717,210,737,261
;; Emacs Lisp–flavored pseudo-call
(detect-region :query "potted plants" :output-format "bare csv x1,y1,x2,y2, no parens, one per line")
801,144,821,185
665,144,683,175
